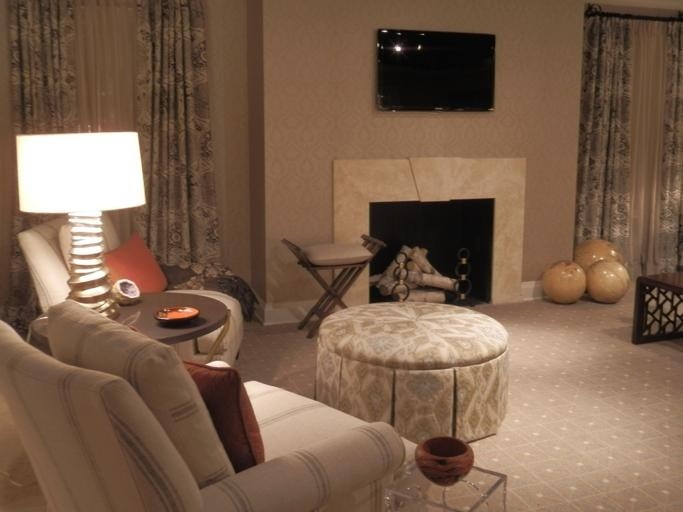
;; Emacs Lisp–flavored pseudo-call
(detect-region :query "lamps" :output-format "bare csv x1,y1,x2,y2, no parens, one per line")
13,129,147,323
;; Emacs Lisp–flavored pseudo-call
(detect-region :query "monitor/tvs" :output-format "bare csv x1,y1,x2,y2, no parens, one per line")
377,28,496,112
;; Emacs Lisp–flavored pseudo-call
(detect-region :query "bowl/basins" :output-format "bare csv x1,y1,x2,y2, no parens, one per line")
413,435,473,486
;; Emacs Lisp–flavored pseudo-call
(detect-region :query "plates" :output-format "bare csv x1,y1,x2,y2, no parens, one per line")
153,305,198,323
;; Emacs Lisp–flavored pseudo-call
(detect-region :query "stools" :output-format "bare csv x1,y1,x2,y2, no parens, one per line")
313,300,511,449
